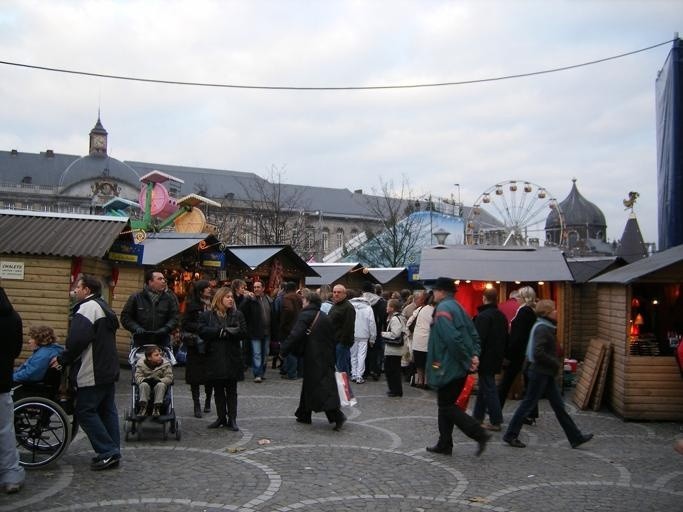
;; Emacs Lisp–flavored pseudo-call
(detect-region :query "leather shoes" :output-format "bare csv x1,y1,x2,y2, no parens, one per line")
569,434,593,448
476,434,490,457
333,414,347,430
480,423,501,431
426,446,452,455
502,435,526,448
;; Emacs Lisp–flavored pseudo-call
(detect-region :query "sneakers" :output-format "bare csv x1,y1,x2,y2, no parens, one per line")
90,454,121,470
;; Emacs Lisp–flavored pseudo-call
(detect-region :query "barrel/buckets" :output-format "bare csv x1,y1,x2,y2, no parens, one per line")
407,264,420,284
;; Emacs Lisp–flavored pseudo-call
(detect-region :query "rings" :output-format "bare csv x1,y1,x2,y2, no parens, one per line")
55,365,62,370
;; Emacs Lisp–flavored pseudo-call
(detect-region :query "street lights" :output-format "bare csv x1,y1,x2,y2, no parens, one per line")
220,191,234,218
454,182,461,203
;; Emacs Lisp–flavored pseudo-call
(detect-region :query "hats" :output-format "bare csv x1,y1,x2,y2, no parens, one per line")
429,278,456,292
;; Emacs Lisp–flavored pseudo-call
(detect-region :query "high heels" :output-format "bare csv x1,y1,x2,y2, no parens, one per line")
208,416,227,428
227,416,238,431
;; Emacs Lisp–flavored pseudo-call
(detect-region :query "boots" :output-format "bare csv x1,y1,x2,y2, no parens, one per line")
136,401,149,416
204,400,210,412
152,403,163,416
194,403,201,418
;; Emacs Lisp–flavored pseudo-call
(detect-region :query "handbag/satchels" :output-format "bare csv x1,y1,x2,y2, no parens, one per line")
384,330,405,347
408,316,418,333
334,372,357,408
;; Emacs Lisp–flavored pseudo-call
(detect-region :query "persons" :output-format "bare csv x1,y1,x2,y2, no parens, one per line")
500,299,594,451
175,274,435,407
194,286,245,433
179,280,216,420
469,285,505,432
277,292,348,433
422,276,493,458
0,285,27,496
130,341,175,418
10,325,65,405
47,275,123,471
118,268,182,338
484,286,538,429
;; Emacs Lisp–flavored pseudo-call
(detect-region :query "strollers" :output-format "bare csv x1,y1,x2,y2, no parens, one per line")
122,330,181,442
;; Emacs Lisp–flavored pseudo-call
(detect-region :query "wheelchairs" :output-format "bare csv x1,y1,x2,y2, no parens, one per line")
10,361,79,469
